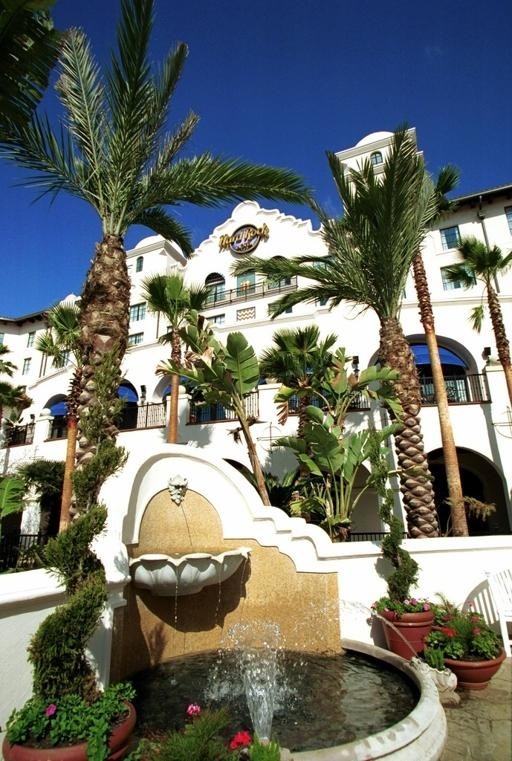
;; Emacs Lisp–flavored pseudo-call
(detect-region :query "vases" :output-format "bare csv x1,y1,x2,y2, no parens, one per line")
442,647,507,692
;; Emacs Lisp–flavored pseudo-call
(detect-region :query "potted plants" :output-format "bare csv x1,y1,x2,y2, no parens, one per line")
362,413,435,661
1,338,137,761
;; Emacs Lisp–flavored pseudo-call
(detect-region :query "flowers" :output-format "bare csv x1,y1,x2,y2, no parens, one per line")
6,681,136,760
423,600,505,664
369,596,441,621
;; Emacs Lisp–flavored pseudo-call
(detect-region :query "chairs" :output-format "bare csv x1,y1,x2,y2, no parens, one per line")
480,558,511,657
